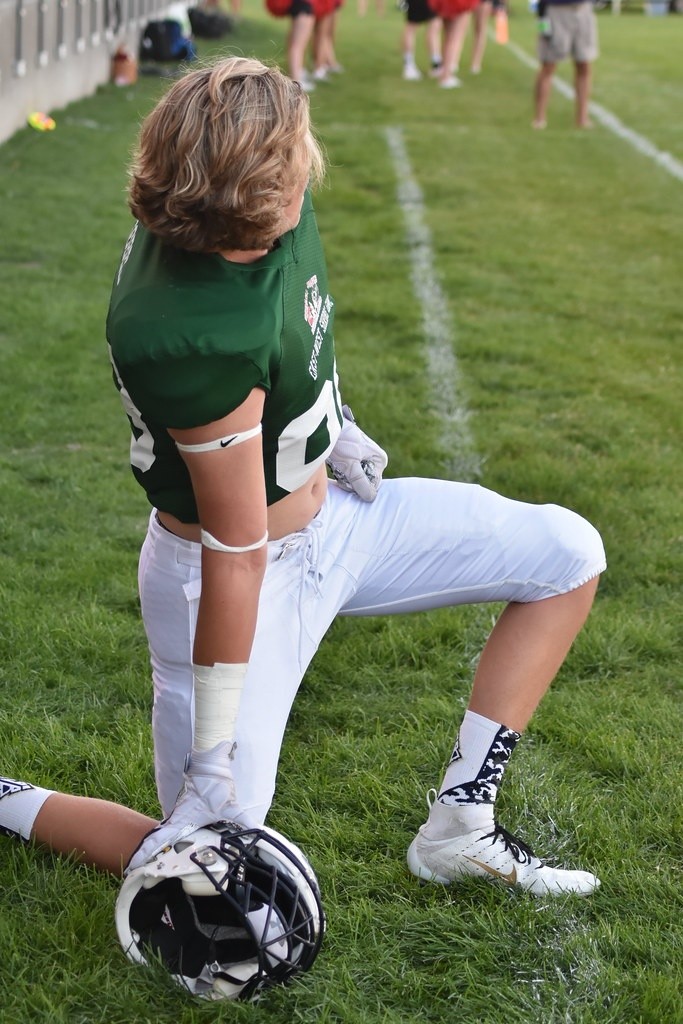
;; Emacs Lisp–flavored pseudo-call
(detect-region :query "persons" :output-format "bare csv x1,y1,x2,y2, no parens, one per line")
267,0,344,92
531,0,610,129
0,57,606,897
402,0,510,90
357,0,385,17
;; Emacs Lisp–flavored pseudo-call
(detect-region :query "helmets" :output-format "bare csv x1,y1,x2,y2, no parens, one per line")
116,821,325,1006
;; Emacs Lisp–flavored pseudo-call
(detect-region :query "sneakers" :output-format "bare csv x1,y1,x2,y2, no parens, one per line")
407,791,602,899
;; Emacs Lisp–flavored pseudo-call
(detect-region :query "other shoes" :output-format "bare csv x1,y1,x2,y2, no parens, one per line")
439,71,457,88
403,63,421,83
294,79,314,94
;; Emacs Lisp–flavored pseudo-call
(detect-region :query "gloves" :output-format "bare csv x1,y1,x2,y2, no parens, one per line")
325,411,388,505
127,740,244,874
